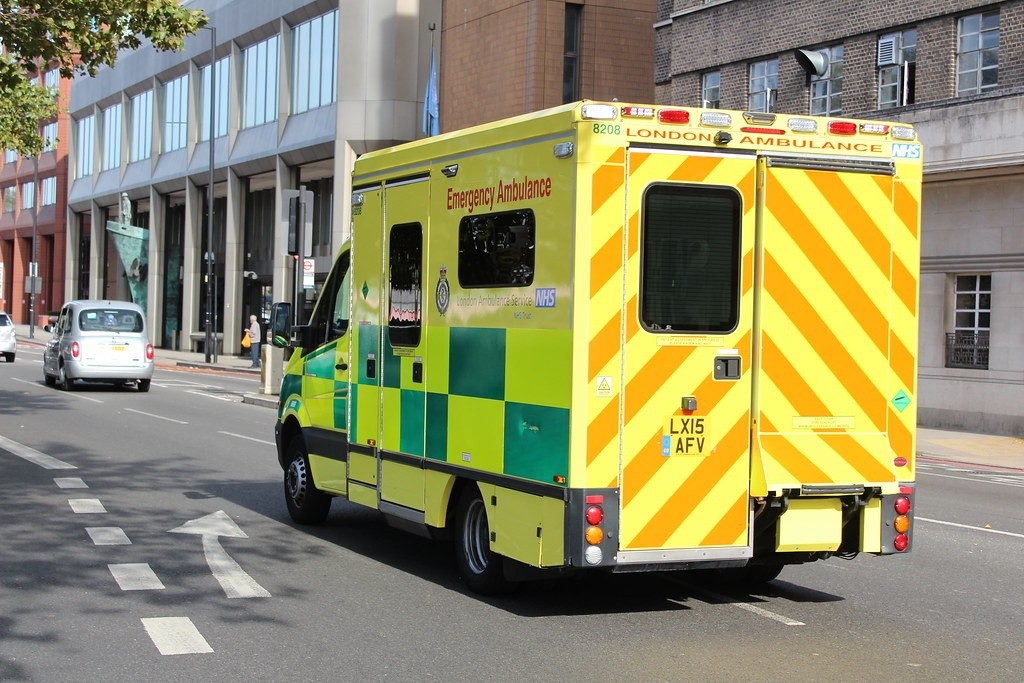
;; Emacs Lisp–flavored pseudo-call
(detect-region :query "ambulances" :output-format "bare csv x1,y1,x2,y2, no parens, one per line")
269,99,925,591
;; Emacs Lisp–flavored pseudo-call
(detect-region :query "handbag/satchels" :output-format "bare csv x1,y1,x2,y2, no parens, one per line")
241,331,251,348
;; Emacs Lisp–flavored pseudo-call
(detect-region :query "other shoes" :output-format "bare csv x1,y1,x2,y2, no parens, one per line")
248,363,260,369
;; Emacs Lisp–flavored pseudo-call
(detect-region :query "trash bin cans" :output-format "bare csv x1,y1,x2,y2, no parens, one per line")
260,345,285,395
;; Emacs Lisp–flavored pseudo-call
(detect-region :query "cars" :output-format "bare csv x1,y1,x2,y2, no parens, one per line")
0,313,16,362
42,300,154,392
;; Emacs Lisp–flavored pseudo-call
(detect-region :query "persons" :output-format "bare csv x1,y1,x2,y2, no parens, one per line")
244,314,261,369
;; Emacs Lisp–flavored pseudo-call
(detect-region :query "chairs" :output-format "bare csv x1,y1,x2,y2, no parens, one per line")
85,313,102,330
120,315,136,330
